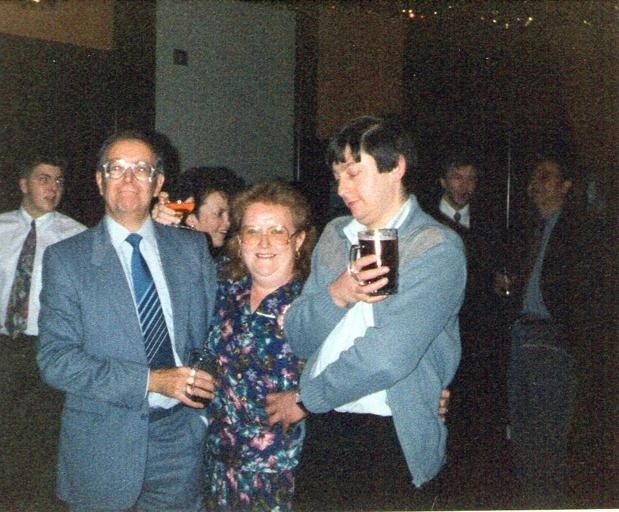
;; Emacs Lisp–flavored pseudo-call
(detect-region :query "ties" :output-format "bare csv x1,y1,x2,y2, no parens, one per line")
125,230,177,372
5,221,37,341
453,211,461,223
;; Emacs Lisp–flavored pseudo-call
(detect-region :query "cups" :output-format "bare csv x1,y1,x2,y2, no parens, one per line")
347,228,401,295
186,348,218,409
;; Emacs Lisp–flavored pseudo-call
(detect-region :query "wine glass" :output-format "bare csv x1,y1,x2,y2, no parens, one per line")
164,190,196,231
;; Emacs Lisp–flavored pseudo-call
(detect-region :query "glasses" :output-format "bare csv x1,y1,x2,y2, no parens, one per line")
101,155,157,185
239,222,307,246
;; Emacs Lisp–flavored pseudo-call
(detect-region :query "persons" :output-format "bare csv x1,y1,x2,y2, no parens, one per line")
263,115,473,511
33,127,221,510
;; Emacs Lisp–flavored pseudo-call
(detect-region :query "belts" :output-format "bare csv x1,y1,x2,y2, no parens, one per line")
149,402,184,424
